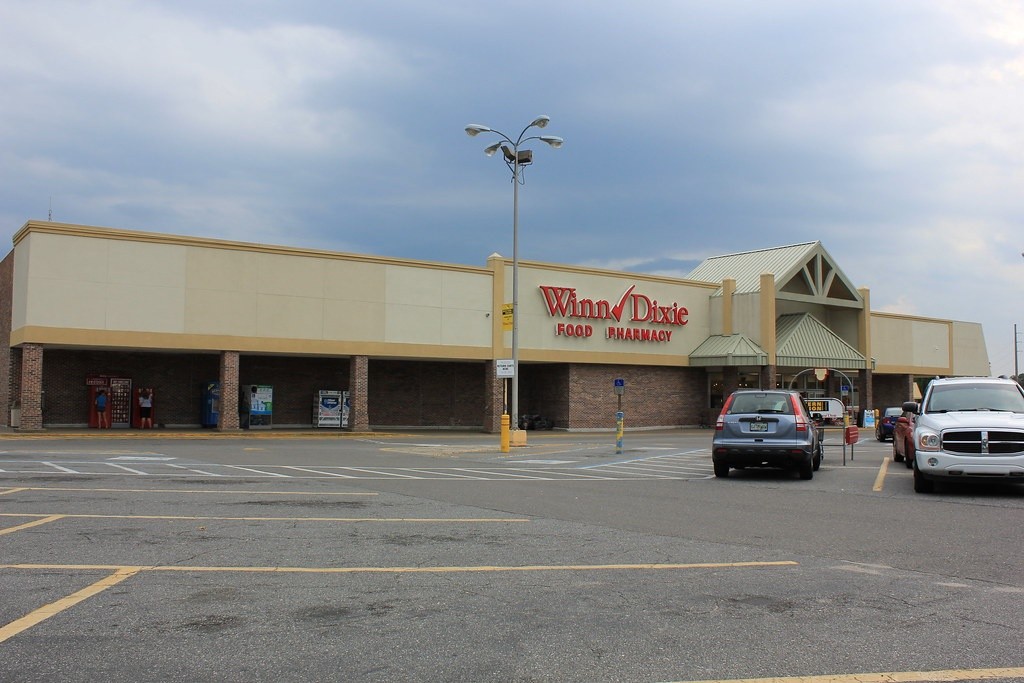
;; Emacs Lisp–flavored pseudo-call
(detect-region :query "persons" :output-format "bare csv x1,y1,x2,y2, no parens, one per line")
95,390,108,429
139,389,153,429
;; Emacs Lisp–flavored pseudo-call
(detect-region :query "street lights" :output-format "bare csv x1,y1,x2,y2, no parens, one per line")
464,114,564,447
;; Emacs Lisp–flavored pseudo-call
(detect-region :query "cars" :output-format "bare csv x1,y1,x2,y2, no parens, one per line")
875,407,904,442
893,403,922,469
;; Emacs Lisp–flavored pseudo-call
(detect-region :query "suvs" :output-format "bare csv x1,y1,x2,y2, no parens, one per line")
902,376,1024,494
711,389,824,481
